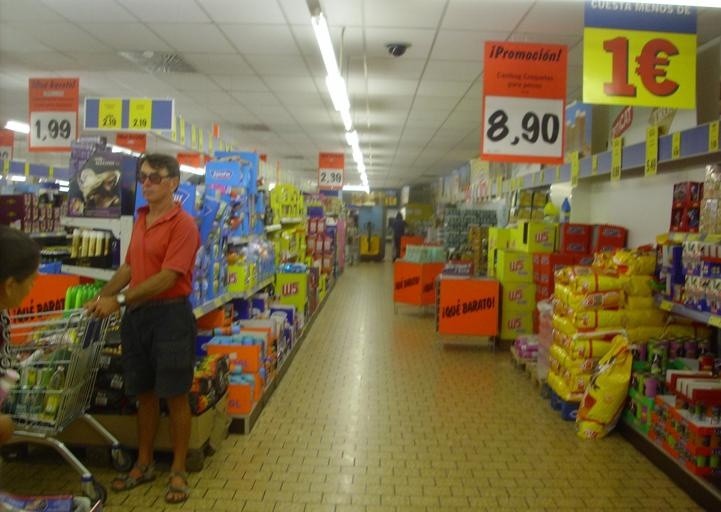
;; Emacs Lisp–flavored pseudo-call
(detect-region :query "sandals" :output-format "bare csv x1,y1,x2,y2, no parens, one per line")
163,469,191,503
109,460,157,494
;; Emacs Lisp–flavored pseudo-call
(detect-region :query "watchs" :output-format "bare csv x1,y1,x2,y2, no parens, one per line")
115,292,128,307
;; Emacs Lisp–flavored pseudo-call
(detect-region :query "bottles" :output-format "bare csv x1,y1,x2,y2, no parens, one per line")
0,368,20,407
38,366,66,421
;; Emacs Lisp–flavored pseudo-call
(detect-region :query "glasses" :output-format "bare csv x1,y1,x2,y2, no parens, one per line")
135,170,176,184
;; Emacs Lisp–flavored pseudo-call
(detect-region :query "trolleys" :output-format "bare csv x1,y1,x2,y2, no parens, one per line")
8,304,134,508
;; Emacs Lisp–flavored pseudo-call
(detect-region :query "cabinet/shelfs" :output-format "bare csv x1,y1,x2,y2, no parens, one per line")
60,215,133,283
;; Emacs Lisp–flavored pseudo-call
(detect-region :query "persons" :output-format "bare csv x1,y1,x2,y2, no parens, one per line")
82,153,197,503
386,212,409,262
0,226,41,442
